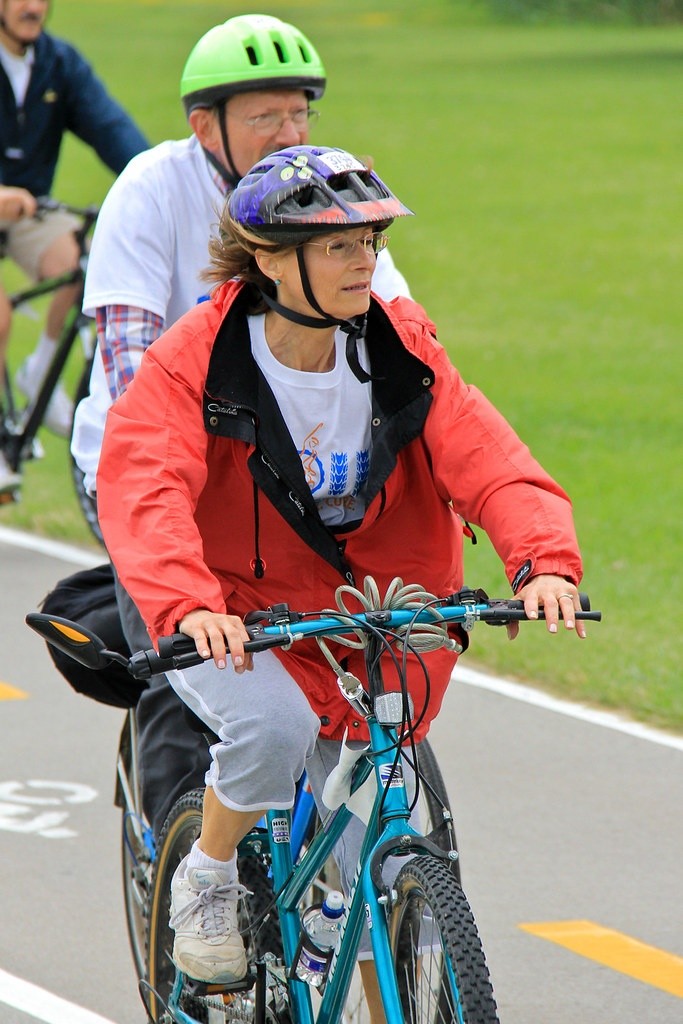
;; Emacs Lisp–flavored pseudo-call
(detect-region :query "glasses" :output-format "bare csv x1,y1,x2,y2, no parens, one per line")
189,103,322,136
303,233,389,260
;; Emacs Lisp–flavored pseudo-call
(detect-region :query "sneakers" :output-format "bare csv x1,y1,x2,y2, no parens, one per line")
169,853,255,984
17,361,75,441
0,450,23,495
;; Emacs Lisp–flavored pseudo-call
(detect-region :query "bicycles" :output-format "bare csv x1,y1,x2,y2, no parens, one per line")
0,193,134,548
24,590,602,1024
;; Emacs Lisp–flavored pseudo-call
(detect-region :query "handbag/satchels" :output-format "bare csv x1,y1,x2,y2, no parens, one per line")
41,562,149,710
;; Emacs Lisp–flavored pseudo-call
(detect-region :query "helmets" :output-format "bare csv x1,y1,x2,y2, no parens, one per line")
179,13,326,121
229,145,414,246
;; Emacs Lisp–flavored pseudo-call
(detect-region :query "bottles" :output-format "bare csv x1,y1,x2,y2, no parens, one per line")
294,890,344,985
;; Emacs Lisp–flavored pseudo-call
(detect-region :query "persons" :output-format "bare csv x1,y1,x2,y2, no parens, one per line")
0,1,158,491
94,144,589,1024
67,12,418,895
